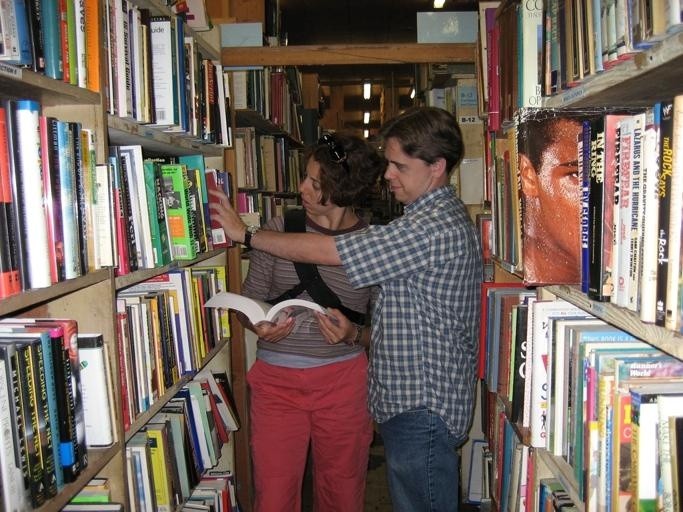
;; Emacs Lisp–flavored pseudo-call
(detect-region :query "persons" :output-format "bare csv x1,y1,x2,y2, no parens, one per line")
209,107,483,512
519,110,584,283
237,132,383,512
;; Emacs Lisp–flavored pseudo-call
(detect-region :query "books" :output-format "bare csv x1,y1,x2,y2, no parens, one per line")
115,264,230,432
1,0,103,95
539,1,682,97
64,478,123,511
238,192,304,248
0,317,117,512
529,300,683,512
99,0,233,149
467,382,532,512
481,282,537,428
0,95,118,301
234,67,303,142
476,209,491,261
370,218,372,219
358,183,404,225
491,128,518,266
513,93,683,336
204,294,338,340
317,126,335,143
484,131,510,202
181,470,238,512
415,64,482,205
485,1,542,131
234,127,303,193
125,366,238,512
109,146,237,277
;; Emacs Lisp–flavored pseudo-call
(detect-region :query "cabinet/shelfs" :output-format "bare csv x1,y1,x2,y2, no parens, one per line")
0,0,308,512
453,0,683,511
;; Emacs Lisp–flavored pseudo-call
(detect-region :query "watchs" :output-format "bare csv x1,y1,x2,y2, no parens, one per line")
244,226,259,249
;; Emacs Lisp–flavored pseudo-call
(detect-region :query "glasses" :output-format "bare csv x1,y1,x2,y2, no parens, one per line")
318,133,350,175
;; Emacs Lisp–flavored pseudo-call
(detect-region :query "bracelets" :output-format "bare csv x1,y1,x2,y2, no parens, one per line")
344,324,362,346
